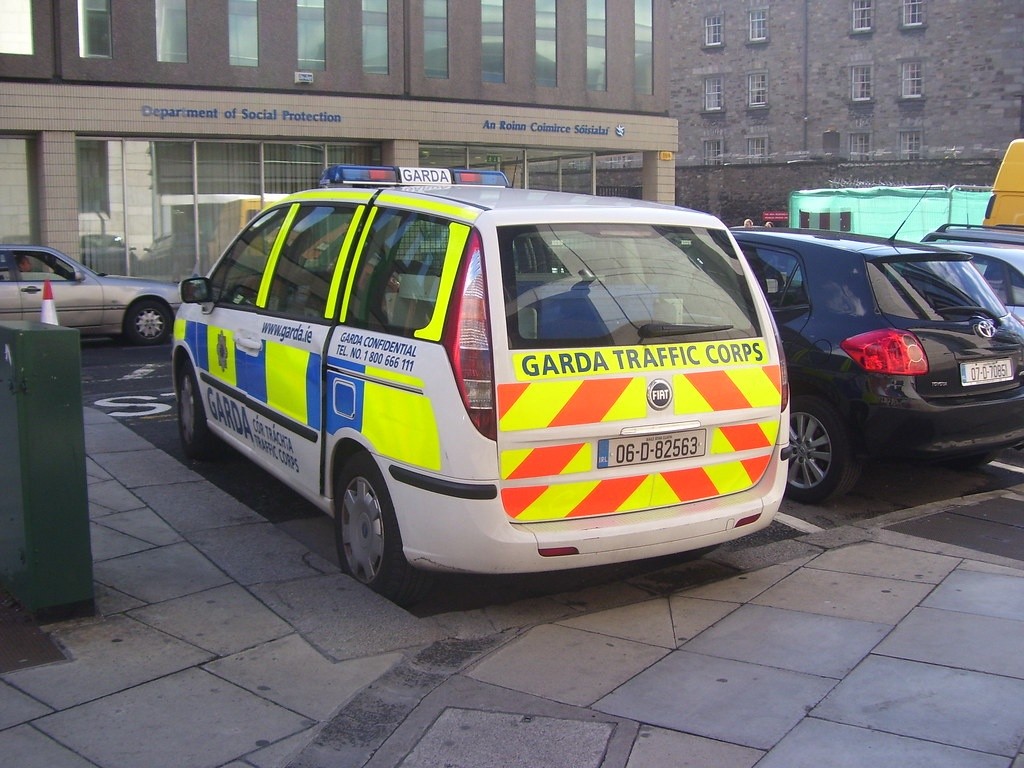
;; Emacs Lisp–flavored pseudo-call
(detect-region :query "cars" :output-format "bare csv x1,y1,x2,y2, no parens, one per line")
0,244,183,346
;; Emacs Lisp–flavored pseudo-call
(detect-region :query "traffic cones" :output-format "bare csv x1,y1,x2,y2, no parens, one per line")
40,279,59,327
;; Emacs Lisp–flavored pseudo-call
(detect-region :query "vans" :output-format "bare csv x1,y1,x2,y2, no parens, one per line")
171,166,792,612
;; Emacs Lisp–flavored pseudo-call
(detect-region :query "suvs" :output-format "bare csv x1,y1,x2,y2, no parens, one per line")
728,224,1024,506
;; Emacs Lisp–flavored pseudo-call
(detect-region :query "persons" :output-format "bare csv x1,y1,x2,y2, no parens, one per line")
744,219,753,226
765,222,774,227
14,254,33,280
